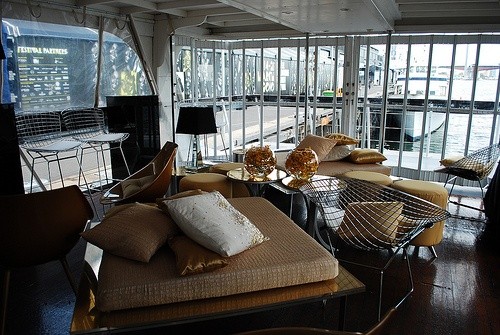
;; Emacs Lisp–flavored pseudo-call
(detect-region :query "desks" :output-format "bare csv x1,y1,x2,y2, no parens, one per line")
227,166,346,239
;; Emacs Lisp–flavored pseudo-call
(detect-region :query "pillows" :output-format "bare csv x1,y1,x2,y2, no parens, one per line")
439,155,490,178
81,175,267,277
295,133,387,164
336,201,418,245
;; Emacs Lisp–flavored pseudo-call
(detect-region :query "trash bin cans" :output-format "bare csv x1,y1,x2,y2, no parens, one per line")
323,91,334,97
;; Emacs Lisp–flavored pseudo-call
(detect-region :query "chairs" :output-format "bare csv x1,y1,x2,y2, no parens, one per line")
434,143,500,222
99,141,178,204
298,176,451,324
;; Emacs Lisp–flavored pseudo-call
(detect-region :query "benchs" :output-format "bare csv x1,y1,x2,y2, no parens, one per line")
71,197,366,335
272,152,391,191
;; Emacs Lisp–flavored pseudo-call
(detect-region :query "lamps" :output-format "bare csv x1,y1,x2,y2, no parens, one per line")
176,106,217,170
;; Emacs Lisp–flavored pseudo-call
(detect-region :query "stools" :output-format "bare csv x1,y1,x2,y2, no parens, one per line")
339,170,392,211
0,185,94,335
391,180,448,259
180,163,252,201
15,106,131,220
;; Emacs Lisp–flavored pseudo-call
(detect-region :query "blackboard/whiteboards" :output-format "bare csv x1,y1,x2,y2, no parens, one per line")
106,95,160,186
